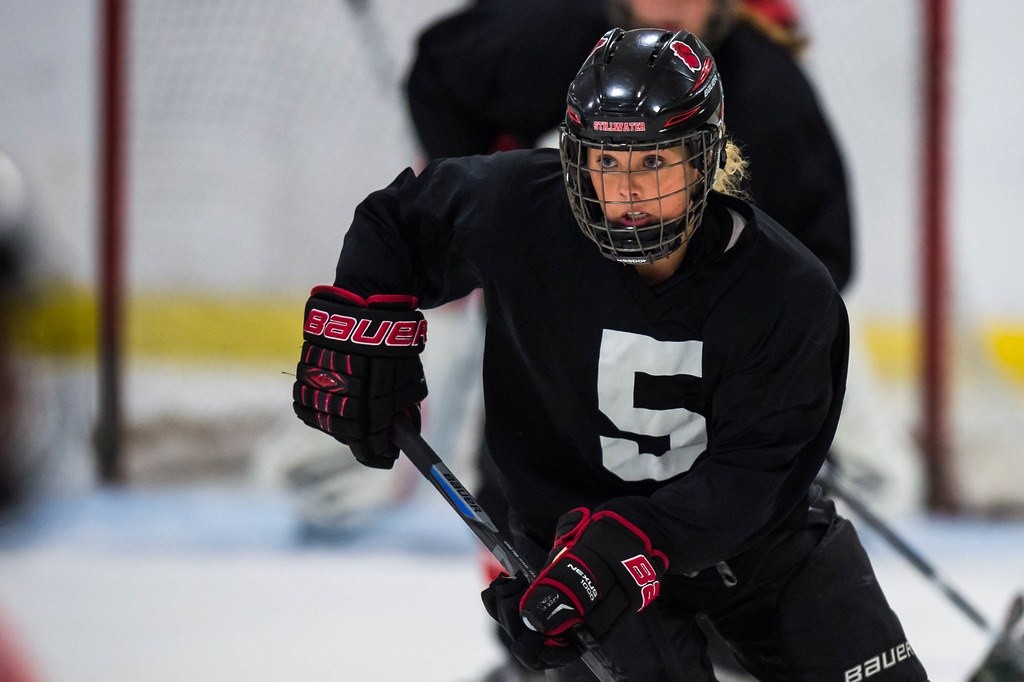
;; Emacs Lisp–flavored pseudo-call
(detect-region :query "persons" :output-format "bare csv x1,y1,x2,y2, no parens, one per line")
285,0,854,533
291,28,929,682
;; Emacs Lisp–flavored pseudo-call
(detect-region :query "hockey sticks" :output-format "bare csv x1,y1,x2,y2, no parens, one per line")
396,434,613,681
818,480,995,637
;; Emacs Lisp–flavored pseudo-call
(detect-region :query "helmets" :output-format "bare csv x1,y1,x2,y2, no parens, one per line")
559,27,726,264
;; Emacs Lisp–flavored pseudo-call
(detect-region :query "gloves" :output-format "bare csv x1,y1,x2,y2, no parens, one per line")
293,286,429,469
481,508,668,669
